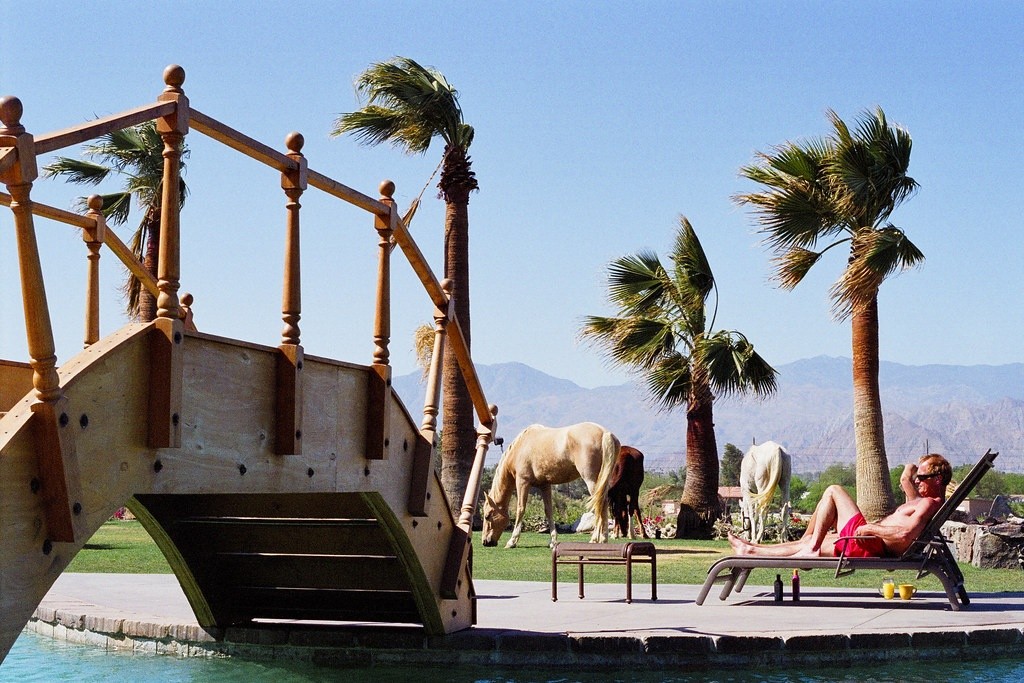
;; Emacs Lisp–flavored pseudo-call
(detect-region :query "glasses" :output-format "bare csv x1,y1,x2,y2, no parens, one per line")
916,472,940,481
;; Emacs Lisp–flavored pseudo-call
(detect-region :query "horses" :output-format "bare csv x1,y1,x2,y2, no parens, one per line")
607,446,652,540
481,422,621,549
738,441,791,545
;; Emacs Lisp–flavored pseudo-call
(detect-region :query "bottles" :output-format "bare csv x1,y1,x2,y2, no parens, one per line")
774,574,783,604
792,569,800,602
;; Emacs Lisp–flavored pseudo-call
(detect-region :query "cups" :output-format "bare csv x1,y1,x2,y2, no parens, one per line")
878,577,894,600
898,584,917,600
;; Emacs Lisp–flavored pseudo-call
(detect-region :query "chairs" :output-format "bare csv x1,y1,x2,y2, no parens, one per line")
696,447,999,611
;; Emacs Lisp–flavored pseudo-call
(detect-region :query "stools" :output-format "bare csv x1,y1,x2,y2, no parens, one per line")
551,540,658,604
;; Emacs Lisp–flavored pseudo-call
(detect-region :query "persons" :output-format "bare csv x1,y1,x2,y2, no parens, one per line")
727,454,955,561
